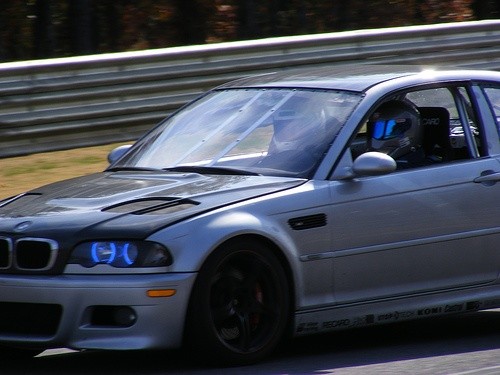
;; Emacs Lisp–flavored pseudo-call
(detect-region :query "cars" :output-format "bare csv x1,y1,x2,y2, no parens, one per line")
0,61,500,368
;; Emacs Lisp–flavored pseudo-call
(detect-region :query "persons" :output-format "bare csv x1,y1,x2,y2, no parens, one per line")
352,97,427,174
261,95,339,171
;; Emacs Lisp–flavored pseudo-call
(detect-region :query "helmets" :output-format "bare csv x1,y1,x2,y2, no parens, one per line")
270,97,327,154
366,98,424,161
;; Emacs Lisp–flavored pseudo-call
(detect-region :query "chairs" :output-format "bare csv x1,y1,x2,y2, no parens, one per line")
267,108,450,166
